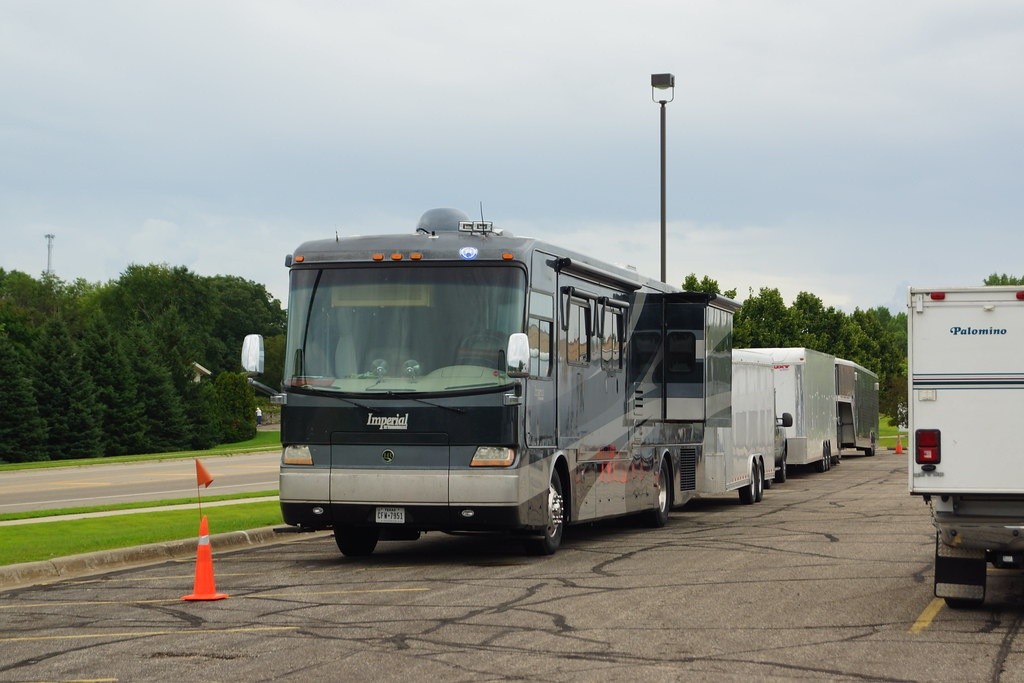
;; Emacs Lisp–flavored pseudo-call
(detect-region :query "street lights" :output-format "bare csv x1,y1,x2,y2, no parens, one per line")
650,72,676,288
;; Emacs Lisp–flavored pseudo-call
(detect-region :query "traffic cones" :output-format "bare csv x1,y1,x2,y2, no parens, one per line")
180,516,229,600
892,432,905,455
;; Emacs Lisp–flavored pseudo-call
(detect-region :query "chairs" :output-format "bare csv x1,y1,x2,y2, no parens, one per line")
633,332,696,375
364,346,407,378
453,331,507,370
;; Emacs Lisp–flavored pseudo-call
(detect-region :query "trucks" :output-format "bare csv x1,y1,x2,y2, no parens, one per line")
905,284,1024,613
731,346,848,474
836,356,881,462
642,347,794,507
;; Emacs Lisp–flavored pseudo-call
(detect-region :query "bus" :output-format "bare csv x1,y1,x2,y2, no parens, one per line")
240,206,742,560
